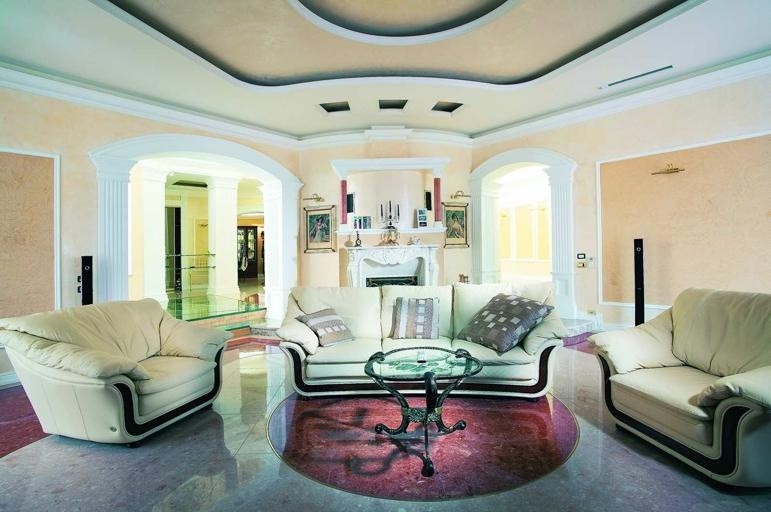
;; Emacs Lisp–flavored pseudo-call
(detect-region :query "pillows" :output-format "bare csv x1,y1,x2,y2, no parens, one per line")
458,292,555,354
393,296,441,341
295,306,355,347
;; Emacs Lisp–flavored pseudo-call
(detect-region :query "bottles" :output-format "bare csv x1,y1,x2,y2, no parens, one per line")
356,234,361,246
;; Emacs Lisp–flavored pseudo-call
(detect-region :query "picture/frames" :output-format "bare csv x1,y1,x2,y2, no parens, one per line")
302,204,338,254
441,201,471,250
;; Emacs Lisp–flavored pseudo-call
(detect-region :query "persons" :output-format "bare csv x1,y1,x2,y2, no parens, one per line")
448,213,463,239
310,217,328,248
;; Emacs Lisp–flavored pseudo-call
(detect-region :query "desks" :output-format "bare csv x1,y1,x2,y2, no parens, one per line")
343,244,438,286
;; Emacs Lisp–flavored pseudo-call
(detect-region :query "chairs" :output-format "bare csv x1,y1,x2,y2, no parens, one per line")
587,286,771,489
0,296,230,446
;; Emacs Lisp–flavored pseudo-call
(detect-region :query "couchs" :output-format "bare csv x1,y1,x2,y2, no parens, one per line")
275,280,568,400
